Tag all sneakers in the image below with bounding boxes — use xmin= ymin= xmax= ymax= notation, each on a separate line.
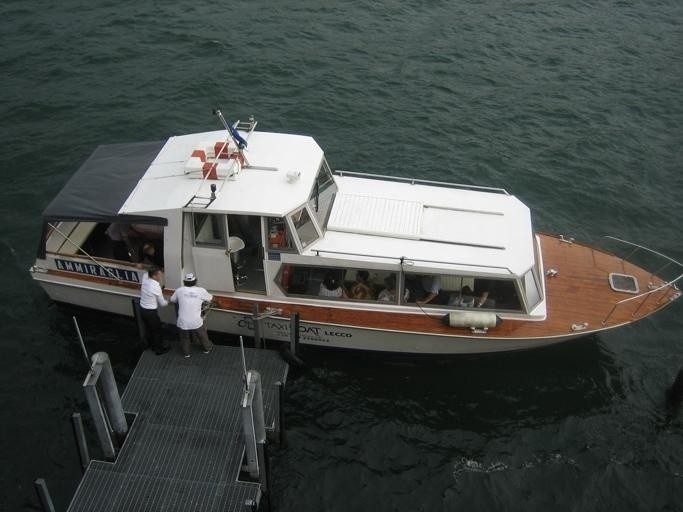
xmin=203 ymin=343 xmax=213 ymax=353
xmin=184 ymin=354 xmax=192 ymax=358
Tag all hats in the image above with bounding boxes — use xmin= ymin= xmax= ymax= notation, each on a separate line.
xmin=183 ymin=273 xmax=196 ymax=282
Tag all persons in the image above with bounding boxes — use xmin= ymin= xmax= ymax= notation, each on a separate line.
xmin=318 ymin=268 xmax=515 ymax=309
xmin=170 ymin=273 xmax=213 ymax=357
xmin=139 ymin=266 xmax=176 ymax=355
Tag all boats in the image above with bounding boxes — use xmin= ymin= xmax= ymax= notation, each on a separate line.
xmin=23 ymin=107 xmax=682 ymax=359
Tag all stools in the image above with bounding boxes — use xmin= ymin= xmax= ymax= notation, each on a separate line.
xmin=228 ymin=236 xmax=247 ymax=287
